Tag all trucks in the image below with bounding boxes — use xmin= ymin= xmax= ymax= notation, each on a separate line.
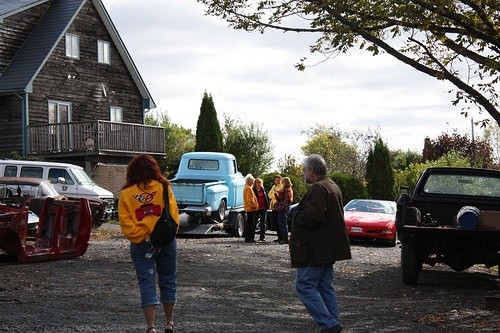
xmin=168 ymin=151 xmax=246 ymax=237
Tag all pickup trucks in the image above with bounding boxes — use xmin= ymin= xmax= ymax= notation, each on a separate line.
xmin=395 ymin=167 xmax=500 ymax=285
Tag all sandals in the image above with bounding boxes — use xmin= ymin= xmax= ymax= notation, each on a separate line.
xmin=163 ymin=323 xmax=173 ymax=333
xmin=145 ymin=327 xmax=157 ymax=333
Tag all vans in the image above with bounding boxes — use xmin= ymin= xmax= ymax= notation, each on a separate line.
xmin=0 ymin=159 xmax=116 ymax=266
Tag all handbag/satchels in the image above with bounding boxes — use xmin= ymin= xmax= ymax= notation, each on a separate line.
xmin=274 ymin=202 xmax=287 ymax=212
xmin=150 ymin=212 xmax=178 ymax=247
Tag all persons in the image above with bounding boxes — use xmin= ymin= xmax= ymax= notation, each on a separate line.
xmin=242 ymin=174 xmax=259 ymax=245
xmin=268 ymin=175 xmax=284 ymax=241
xmin=118 ymin=154 xmax=179 ymax=333
xmin=252 ymin=178 xmax=270 ymax=241
xmin=289 ymin=154 xmax=352 ymax=333
xmin=273 ymin=177 xmax=293 ymax=243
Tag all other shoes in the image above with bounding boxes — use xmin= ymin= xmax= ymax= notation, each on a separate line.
xmin=274 ymin=239 xmax=281 ymax=242
xmin=320 ymin=324 xmax=342 ymax=333
xmin=279 ymin=240 xmax=289 ymax=244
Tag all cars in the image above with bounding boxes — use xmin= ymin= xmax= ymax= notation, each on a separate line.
xmin=343 ymin=198 xmax=401 ymax=247
xmin=255 ymin=202 xmax=299 ymax=234
